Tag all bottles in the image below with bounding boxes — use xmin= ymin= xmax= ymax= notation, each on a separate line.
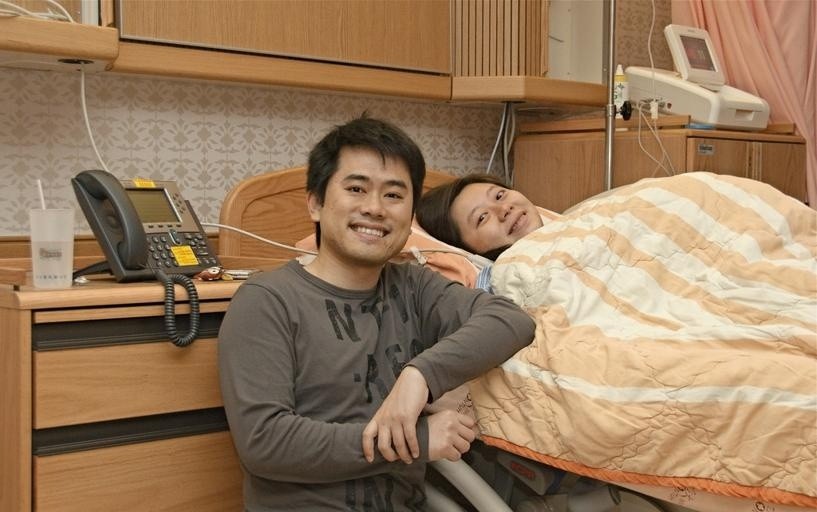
xmin=612 ymin=63 xmax=628 ymax=134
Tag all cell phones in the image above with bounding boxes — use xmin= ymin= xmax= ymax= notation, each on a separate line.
xmin=223 ymin=268 xmax=260 ymax=280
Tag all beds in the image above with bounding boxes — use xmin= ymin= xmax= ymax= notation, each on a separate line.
xmin=210 ymin=162 xmax=817 ymax=512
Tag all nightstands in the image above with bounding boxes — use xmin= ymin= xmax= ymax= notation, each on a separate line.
xmin=0 ymin=254 xmax=252 ymax=511
xmin=514 ymin=118 xmax=807 ymax=213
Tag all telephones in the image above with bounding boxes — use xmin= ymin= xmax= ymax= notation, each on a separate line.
xmin=71 ymin=169 xmax=224 ymax=283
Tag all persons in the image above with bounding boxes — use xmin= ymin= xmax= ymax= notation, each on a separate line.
xmin=216 ymin=113 xmax=537 ymax=512
xmin=414 ymin=174 xmax=544 ymax=258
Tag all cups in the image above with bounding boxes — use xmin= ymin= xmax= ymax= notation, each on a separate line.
xmin=26 ymin=206 xmax=78 ymax=291
